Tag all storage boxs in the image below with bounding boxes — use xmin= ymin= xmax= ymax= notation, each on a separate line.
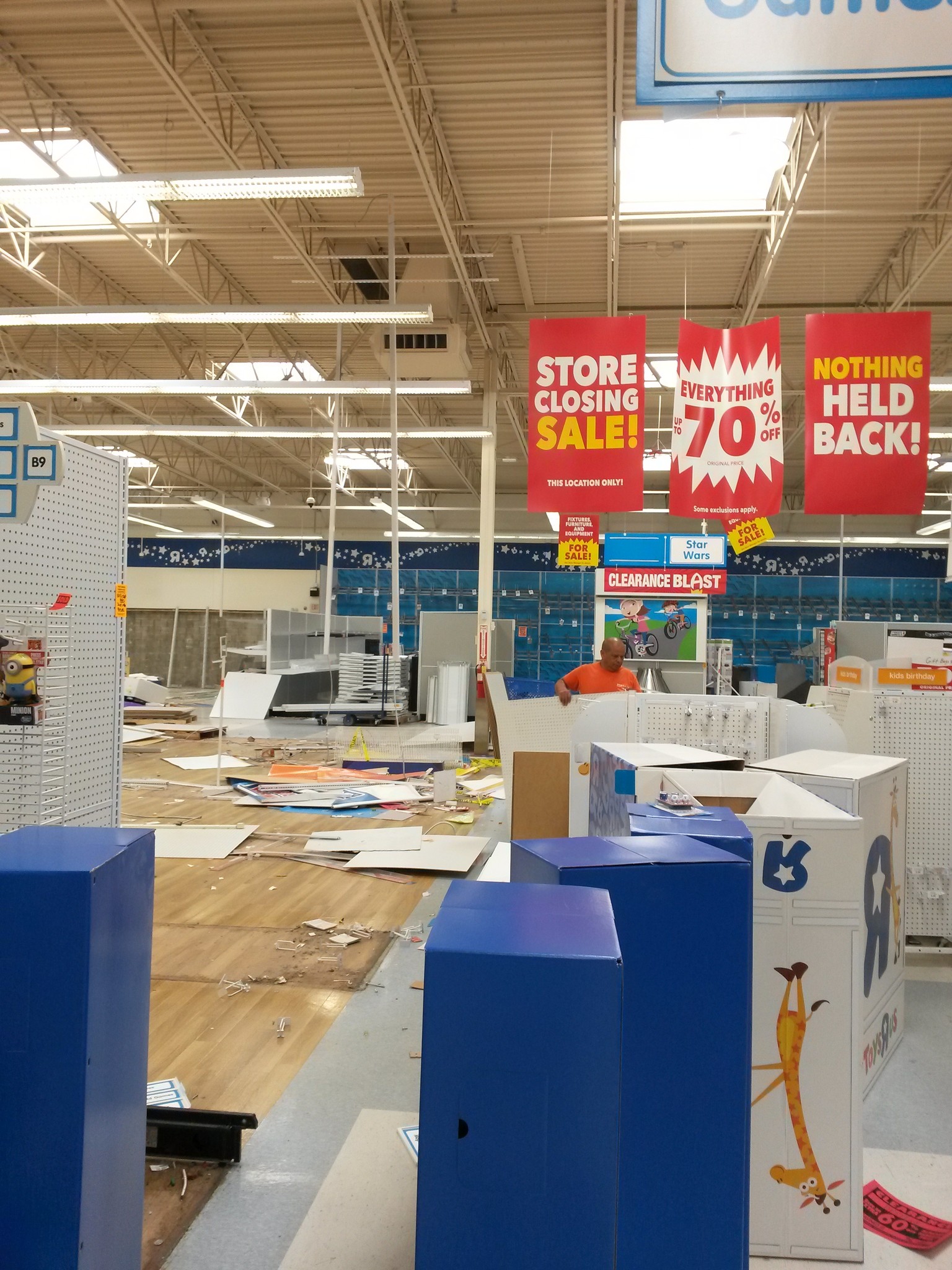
xmin=415 ymin=742 xmax=909 ymax=1270
xmin=0 ymin=823 xmax=156 ymax=1269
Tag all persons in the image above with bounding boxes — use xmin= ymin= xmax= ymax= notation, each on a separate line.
xmin=554 ymin=638 xmax=642 ymax=706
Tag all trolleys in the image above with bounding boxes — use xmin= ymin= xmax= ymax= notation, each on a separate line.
xmin=316 ymin=641 xmax=389 ymax=726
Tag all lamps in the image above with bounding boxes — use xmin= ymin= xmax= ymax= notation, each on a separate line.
xmin=0 ymin=169 xmax=367 ymax=206
xmin=0 ymin=304 xmax=493 ymax=440
xmin=368 ymin=496 xmax=426 ymax=532
xmin=188 ymin=494 xmax=275 ymax=529
xmin=916 ymin=518 xmax=952 ymax=537
xmin=127 ymin=512 xmax=183 ymax=533
xmin=928 ymin=376 xmax=952 ymax=392
xmin=928 ymin=426 xmax=952 ymax=438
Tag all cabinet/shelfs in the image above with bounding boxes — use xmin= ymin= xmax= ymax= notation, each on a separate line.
xmin=333 ymin=586 xmax=952 ymax=664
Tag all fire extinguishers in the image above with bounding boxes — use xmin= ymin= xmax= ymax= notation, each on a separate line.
xmin=475 ymin=662 xmax=486 ymax=699
